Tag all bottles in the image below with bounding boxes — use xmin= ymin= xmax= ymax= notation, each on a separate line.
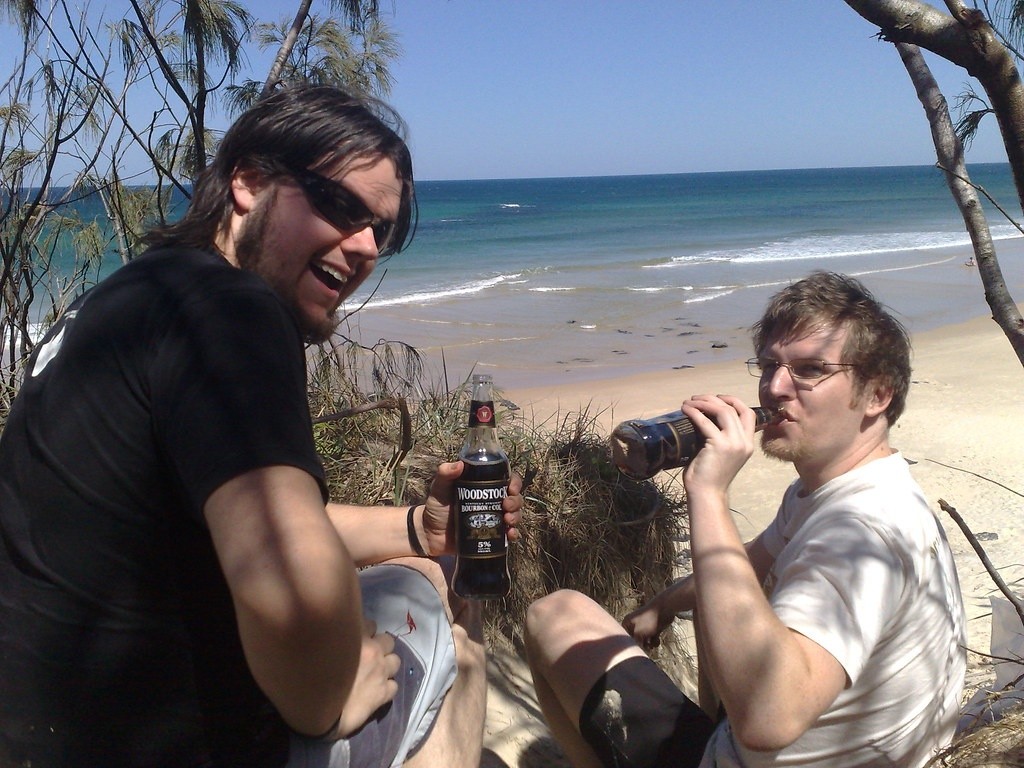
xmin=609 ymin=404 xmax=778 ymax=484
xmin=450 ymin=373 xmax=514 ymax=603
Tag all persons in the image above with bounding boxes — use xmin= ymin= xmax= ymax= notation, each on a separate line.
xmin=522 ymin=270 xmax=967 ymax=768
xmin=0 ymin=85 xmax=522 ymax=768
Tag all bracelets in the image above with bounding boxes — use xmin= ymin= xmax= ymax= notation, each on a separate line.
xmin=290 ymin=713 xmax=342 ymax=739
xmin=407 ymin=504 xmax=428 ymax=558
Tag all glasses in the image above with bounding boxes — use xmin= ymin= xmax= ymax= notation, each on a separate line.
xmin=277 ymin=158 xmax=399 ymax=251
xmin=745 ymin=356 xmax=853 ymax=379
